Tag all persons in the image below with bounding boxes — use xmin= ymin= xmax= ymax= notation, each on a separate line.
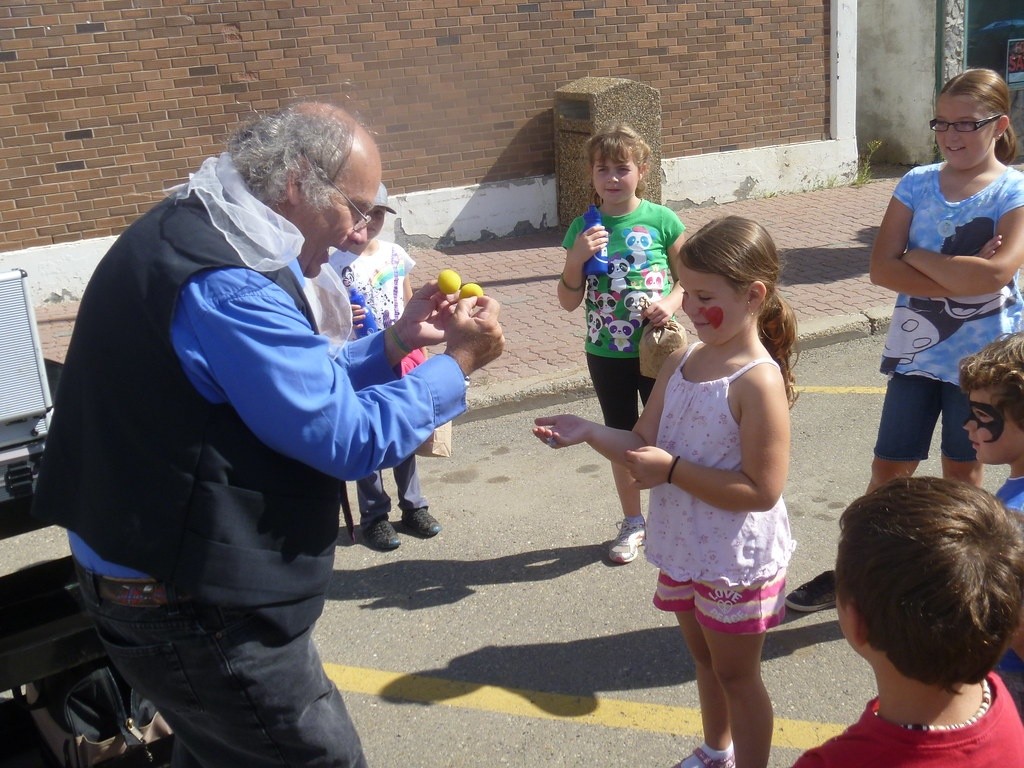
xmin=32 ymin=100 xmax=506 ymax=768
xmin=327 ymin=182 xmax=443 ymax=553
xmin=783 ymin=69 xmax=1024 ymax=613
xmin=557 ymin=124 xmax=687 ymax=564
xmin=531 ymin=213 xmax=799 ymax=768
xmin=789 ymin=333 xmax=1024 ymax=768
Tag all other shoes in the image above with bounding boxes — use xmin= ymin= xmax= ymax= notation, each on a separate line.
xmin=672 ymin=747 xmax=735 ymax=768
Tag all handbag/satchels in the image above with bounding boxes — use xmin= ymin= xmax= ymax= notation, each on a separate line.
xmin=26 ymin=662 xmax=174 ymax=768
xmin=415 ymin=419 xmax=452 ymax=457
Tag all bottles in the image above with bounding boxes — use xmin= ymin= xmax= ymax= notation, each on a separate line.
xmin=582 ymin=205 xmax=608 ymax=274
xmin=349 ymin=287 xmax=376 ymax=341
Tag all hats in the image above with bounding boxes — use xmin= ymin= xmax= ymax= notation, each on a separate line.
xmin=373 ymin=182 xmax=396 ymax=216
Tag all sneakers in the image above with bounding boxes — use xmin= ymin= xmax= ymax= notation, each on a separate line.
xmin=785 ymin=571 xmax=837 ymax=610
xmin=362 ymin=519 xmax=401 ymax=549
xmin=608 ymin=519 xmax=647 ymax=562
xmin=402 ymin=508 xmax=442 ymax=536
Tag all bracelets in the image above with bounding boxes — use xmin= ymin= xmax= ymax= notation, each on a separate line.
xmin=447 ymin=354 xmax=471 ymax=391
xmin=560 ymin=273 xmax=583 ymax=292
xmin=667 ymin=455 xmax=681 ymax=485
xmin=387 ymin=327 xmax=413 ymax=356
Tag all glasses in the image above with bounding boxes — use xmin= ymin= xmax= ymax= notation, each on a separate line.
xmin=300 ymin=148 xmax=372 ymax=232
xmin=930 ymin=113 xmax=1004 ymax=132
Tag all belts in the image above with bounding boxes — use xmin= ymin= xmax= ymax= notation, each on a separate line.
xmin=73 ymin=563 xmax=167 ymax=610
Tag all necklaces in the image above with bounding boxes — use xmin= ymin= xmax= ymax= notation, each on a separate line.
xmin=900 ymin=676 xmax=993 ymax=732
